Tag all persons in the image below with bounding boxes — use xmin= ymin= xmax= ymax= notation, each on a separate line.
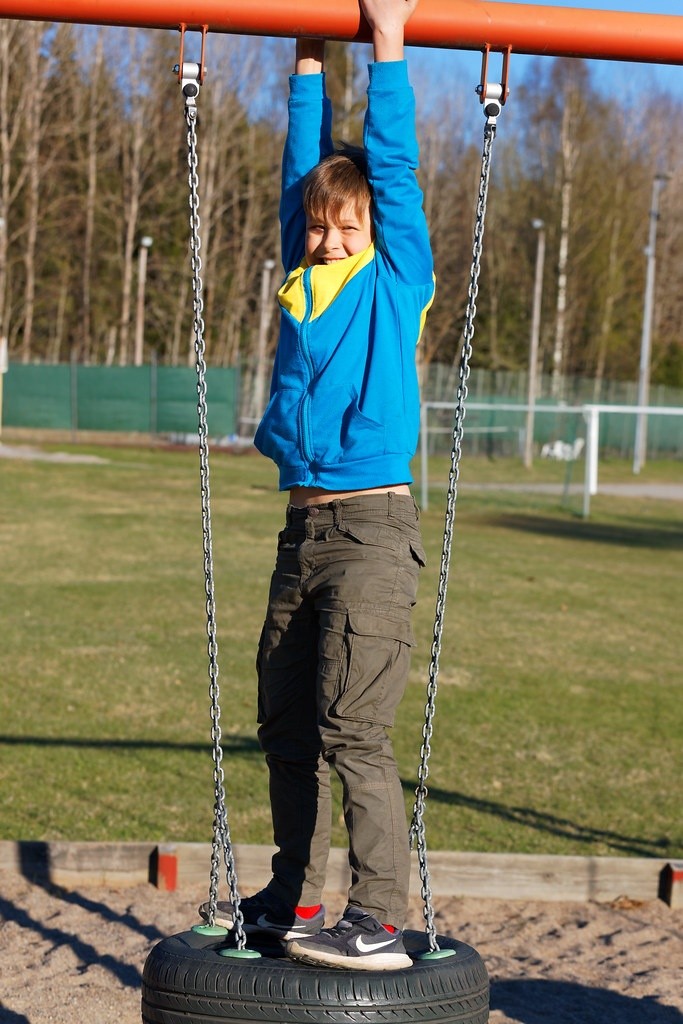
xmin=199 ymin=0 xmax=441 ymax=971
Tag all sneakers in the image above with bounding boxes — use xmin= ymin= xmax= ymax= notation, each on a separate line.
xmin=286 ymin=906 xmax=412 ymax=972
xmin=198 ymin=883 xmax=325 ymax=941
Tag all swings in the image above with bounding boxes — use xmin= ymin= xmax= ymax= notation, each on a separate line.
xmin=142 ymin=23 xmax=514 ymax=1024
